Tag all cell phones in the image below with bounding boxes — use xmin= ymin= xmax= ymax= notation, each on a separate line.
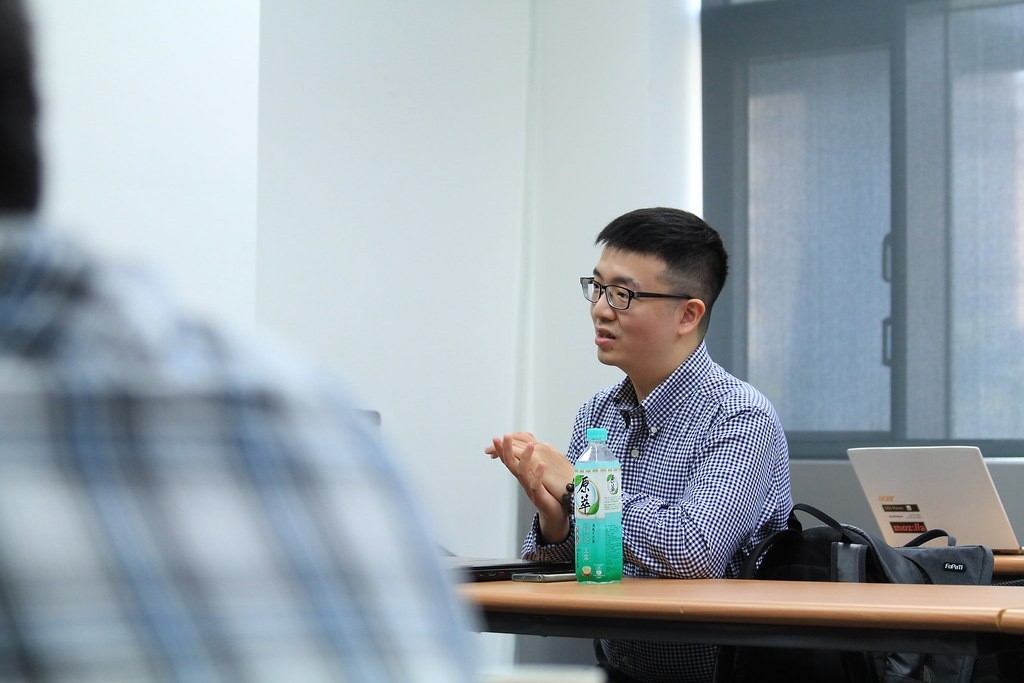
xmin=512 ymin=573 xmax=576 ymax=582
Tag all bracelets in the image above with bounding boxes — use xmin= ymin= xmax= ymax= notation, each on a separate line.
xmin=559 ymin=475 xmax=578 ymax=512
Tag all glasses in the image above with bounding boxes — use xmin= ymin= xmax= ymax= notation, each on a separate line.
xmin=580 ymin=276 xmax=707 ymax=312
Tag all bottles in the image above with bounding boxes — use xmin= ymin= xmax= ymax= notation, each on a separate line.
xmin=574 ymin=429 xmax=623 ymax=583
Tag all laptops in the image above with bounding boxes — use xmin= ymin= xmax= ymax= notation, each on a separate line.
xmin=442 ymin=555 xmax=575 ymax=584
xmin=846 ymin=446 xmax=1024 ymax=555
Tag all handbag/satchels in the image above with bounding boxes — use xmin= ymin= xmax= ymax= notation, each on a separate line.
xmin=713 ymin=503 xmax=994 ymax=683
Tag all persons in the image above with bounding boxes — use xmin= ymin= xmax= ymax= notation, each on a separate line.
xmin=0 ymin=0 xmax=483 ymax=682
xmin=484 ymin=206 xmax=795 ymax=683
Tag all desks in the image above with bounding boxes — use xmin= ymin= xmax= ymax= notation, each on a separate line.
xmin=454 ymin=578 xmax=1023 ymax=682
